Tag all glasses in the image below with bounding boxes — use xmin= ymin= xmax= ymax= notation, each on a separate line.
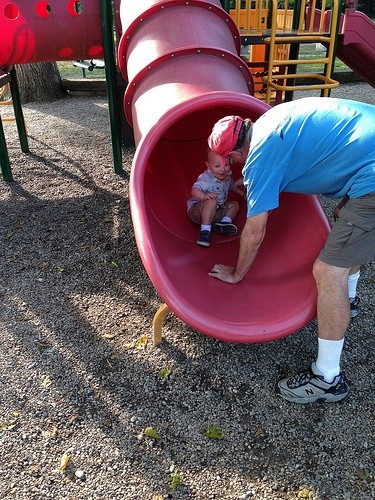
xmin=227 ymin=156 xmax=235 ymax=166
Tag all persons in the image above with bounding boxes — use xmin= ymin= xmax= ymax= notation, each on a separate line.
xmin=186 ymin=149 xmax=248 ymax=247
xmin=209 ymin=97 xmax=375 ymax=405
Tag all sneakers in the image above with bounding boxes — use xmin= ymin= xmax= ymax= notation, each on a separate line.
xmin=275 ymin=362 xmax=348 ymax=402
xmin=197 ymin=230 xmax=211 ymax=247
xmin=348 ymin=297 xmax=361 ymax=318
xmin=213 ymin=221 xmax=238 ymax=236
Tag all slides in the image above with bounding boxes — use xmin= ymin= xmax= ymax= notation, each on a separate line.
xmin=112 ymin=0 xmax=335 ymax=344
xmin=304 ymin=6 xmax=375 ymax=90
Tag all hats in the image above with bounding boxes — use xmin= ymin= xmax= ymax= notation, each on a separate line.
xmin=207 ymin=115 xmax=243 ymax=167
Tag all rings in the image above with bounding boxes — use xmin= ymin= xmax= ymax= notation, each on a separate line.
xmin=217 ymin=269 xmax=218 ymax=272
